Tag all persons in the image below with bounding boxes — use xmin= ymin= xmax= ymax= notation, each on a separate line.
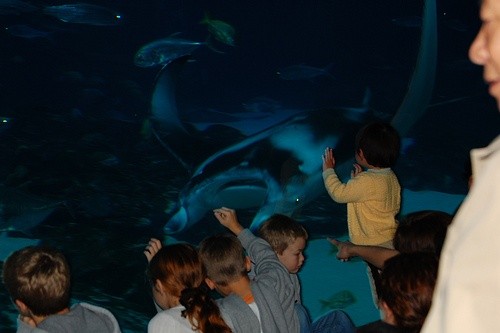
xmin=326 ymin=208 xmax=452 ymax=284
xmin=417 ymin=1 xmax=499 ymax=332
xmin=254 ymin=212 xmax=359 ymax=332
xmin=352 ymin=255 xmax=436 ymax=332
xmin=320 ymin=122 xmax=403 ymax=320
xmin=0 ymin=243 xmax=121 ymax=332
xmin=146 ymin=242 xmax=232 ymax=332
xmin=142 ymin=205 xmax=300 ymax=332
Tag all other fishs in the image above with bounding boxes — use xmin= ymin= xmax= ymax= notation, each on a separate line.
xmin=1 ymin=0 xmax=468 ymax=262
